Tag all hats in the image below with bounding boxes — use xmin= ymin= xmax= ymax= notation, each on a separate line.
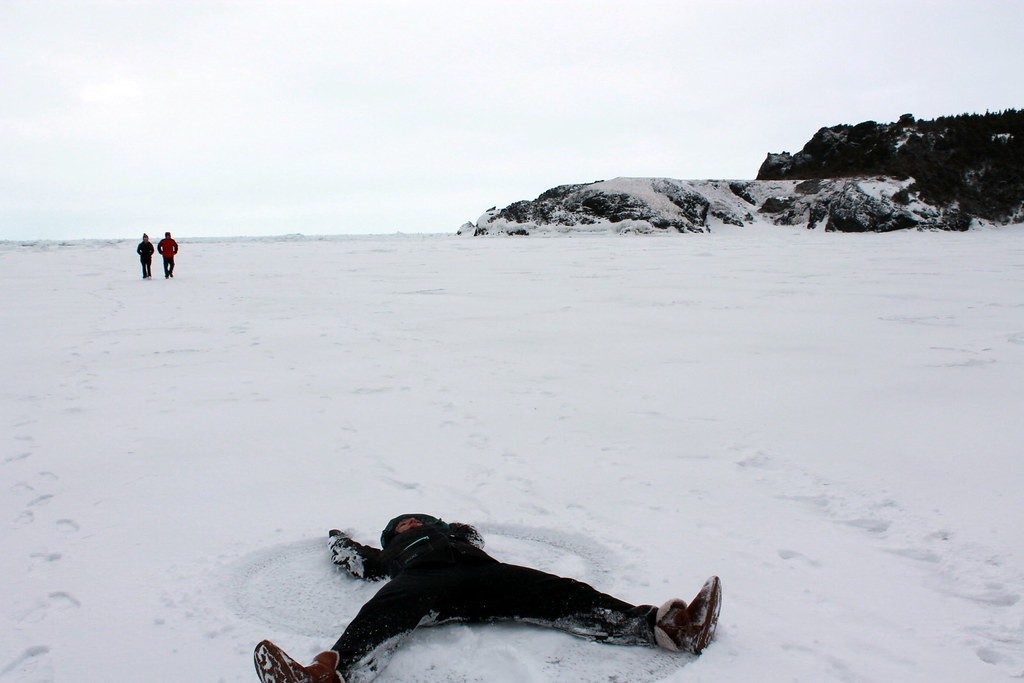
xmin=142 ymin=233 xmax=147 ymax=237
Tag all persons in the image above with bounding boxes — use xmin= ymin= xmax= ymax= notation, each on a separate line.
xmin=255 ymin=514 xmax=722 ymax=683
xmin=158 ymin=232 xmax=178 ymax=279
xmin=137 ymin=234 xmax=155 ymax=279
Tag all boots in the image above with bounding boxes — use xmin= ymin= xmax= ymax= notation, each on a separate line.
xmin=254 ymin=640 xmax=348 ymax=683
xmin=656 ymin=576 xmax=722 ymax=653
